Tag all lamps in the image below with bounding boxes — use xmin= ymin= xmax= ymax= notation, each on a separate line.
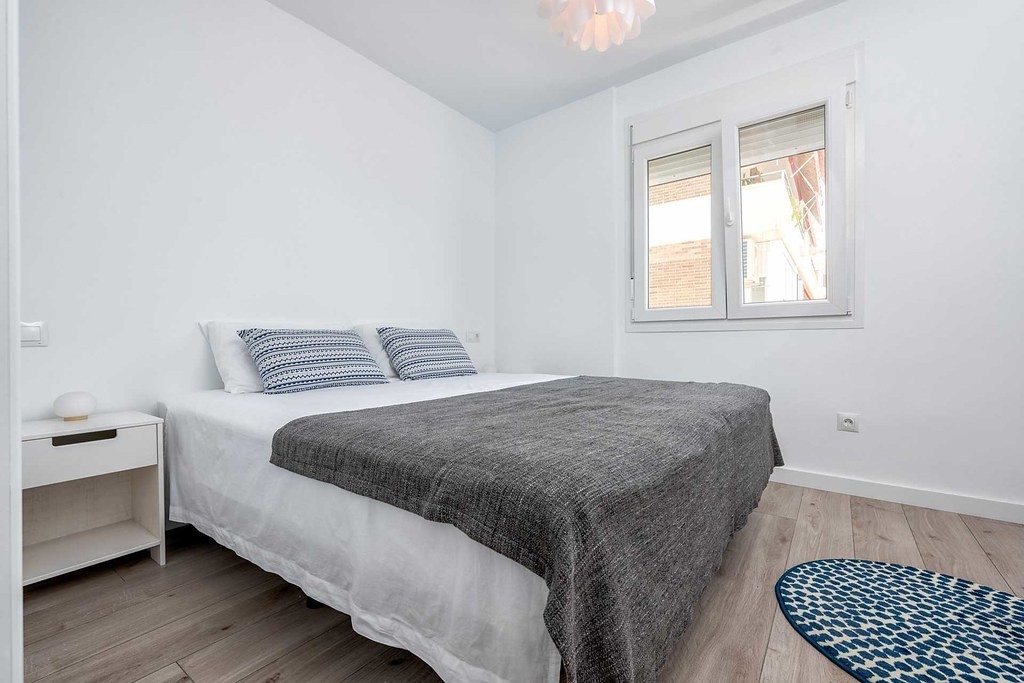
xmin=55 ymin=390 xmax=99 ymax=422
xmin=536 ymin=0 xmax=657 ymax=52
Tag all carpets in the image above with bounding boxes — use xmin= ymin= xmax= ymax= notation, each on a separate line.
xmin=774 ymin=558 xmax=1024 ymax=683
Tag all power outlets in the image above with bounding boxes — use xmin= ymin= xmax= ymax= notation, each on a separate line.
xmin=836 ymin=412 xmax=860 ymax=433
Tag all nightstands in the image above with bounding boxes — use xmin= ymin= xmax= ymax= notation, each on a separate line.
xmin=21 ymin=411 xmax=166 ymax=592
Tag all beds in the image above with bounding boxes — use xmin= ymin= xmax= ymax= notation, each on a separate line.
xmin=153 ymin=313 xmax=786 ymax=683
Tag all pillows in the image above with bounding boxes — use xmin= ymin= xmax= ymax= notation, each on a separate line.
xmin=236 ymin=329 xmax=389 ymax=393
xmin=356 ymin=322 xmax=465 ymax=378
xmin=376 ymin=327 xmax=478 ymax=382
xmin=198 ymin=320 xmax=357 ymax=394
